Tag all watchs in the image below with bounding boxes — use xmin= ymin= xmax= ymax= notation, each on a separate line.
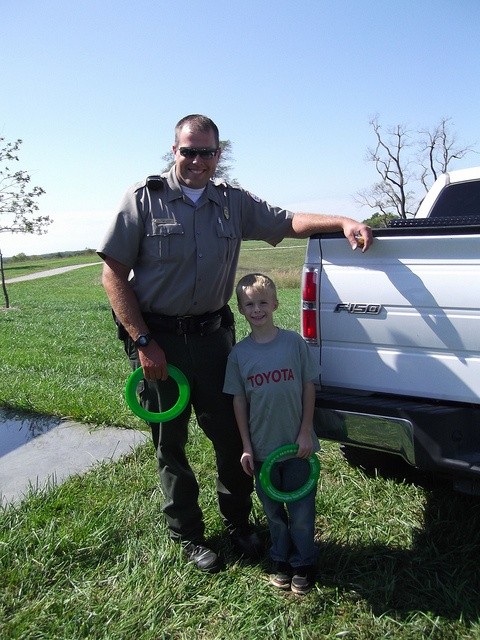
xmin=134 ymin=334 xmax=154 ymax=351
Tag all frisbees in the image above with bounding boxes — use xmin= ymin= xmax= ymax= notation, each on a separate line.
xmin=259 ymin=443 xmax=320 ymax=502
xmin=124 ymin=361 xmax=190 ymax=423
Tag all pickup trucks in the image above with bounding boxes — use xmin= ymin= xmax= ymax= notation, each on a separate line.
xmin=302 ymin=167 xmax=480 ymax=494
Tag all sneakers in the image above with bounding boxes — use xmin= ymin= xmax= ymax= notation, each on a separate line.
xmin=291 ymin=566 xmax=313 ymax=594
xmin=270 ymin=561 xmax=292 ymax=588
xmin=190 ymin=546 xmax=221 ymax=572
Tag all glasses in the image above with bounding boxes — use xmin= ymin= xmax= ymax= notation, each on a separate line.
xmin=178 ymin=147 xmax=221 ymax=159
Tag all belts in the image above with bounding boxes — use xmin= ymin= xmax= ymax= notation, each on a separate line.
xmin=143 ymin=314 xmax=222 ymax=337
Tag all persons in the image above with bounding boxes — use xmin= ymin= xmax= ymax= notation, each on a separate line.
xmin=222 ymin=273 xmax=323 ymax=597
xmin=95 ymin=114 xmax=373 ymax=573
